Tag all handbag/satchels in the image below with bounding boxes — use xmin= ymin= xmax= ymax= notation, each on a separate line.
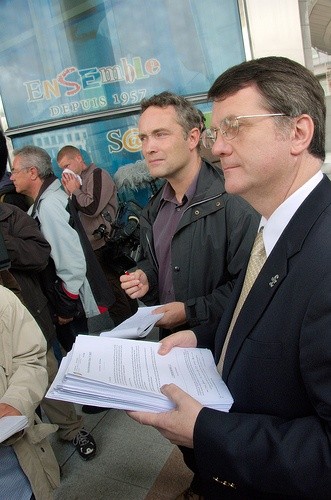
xmin=92 ymin=198 xmax=150 ymax=265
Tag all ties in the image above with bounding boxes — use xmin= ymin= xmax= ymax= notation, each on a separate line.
xmin=216 ymin=229 xmax=267 ymax=378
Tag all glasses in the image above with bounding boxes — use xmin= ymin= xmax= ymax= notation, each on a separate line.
xmin=198 ymin=113 xmax=288 ymax=150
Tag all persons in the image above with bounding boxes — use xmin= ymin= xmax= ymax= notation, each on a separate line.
xmin=0 ymin=203 xmax=97 ymax=458
xmin=9 ymin=145 xmax=119 ymax=414
xmin=0 ymin=284 xmax=63 ymax=500
xmin=0 ymin=91 xmax=259 ymax=340
xmin=124 ymin=55 xmax=331 ymax=500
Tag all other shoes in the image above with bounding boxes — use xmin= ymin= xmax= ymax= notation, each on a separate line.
xmin=81 ymin=404 xmax=109 ymax=414
xmin=70 ymin=427 xmax=96 ymax=461
